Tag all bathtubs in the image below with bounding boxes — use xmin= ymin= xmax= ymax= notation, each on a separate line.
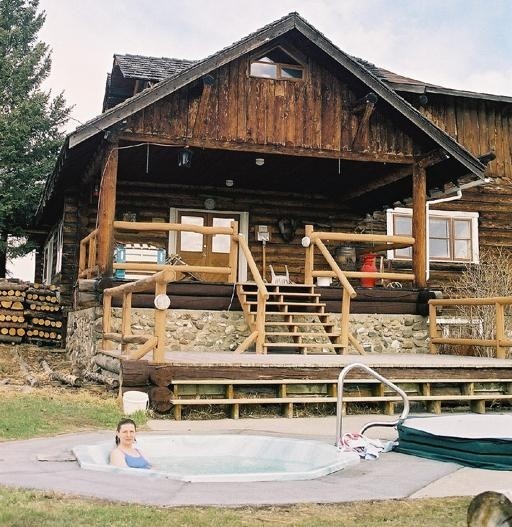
xmin=71 ymin=434 xmax=363 ymax=482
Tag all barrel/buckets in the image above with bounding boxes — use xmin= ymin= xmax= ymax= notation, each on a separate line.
xmin=122 ymin=390 xmax=149 ymax=417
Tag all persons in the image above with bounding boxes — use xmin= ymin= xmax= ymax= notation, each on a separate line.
xmin=109 ymin=417 xmax=159 ymax=471
